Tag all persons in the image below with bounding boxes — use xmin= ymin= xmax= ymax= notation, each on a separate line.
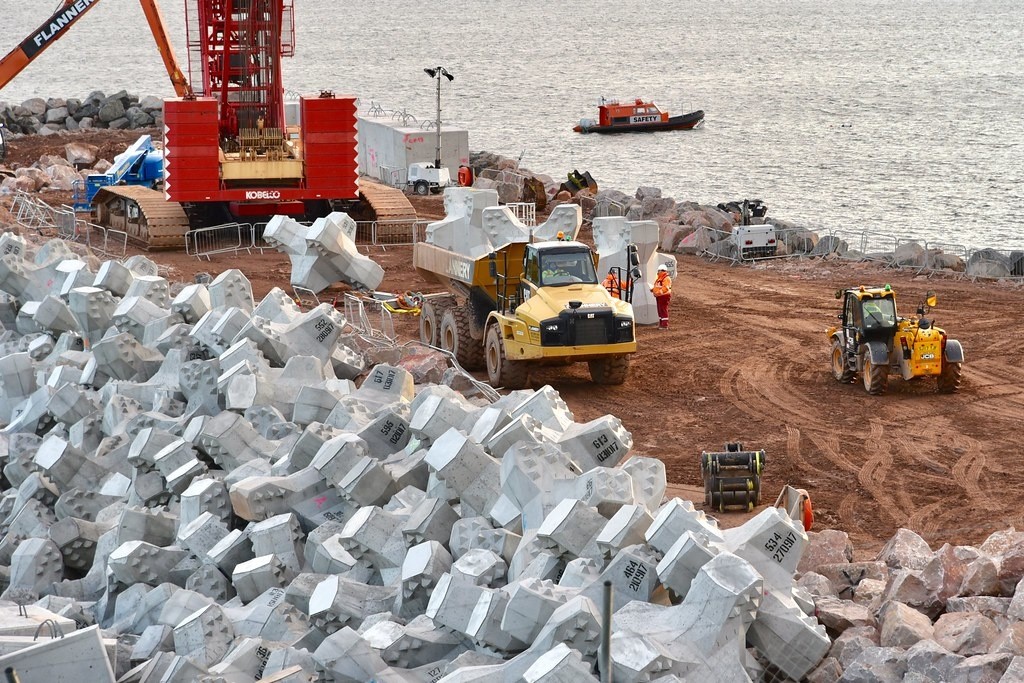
xmin=542 ymin=261 xmax=570 ymax=282
xmin=650 ymin=264 xmax=672 ymax=331
xmin=600 ymin=269 xmax=632 ymax=299
xmin=863 ymin=300 xmax=881 ymax=318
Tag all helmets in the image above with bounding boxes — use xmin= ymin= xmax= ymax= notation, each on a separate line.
xmin=608 ymin=269 xmax=616 ymax=274
xmin=658 ymin=264 xmax=668 ymax=271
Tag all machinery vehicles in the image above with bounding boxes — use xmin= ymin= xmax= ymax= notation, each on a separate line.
xmin=71 ymin=135 xmax=164 ymax=213
xmin=412 ymin=231 xmax=641 ymax=389
xmin=717 ymin=198 xmax=777 ymax=265
xmin=0 ymin=0 xmax=418 ymax=252
xmin=407 ymin=65 xmax=484 ymax=197
xmin=824 ymin=284 xmax=964 ymax=396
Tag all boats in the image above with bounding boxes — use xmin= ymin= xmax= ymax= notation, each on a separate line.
xmin=572 ymin=95 xmax=705 ymax=135
